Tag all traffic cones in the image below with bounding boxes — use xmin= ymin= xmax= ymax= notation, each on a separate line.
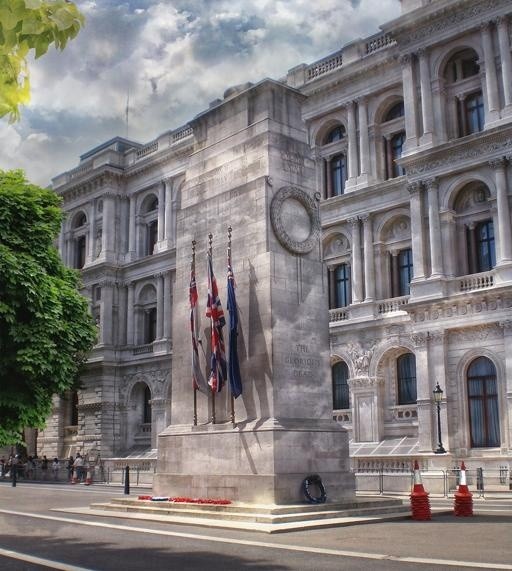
xmin=85 ymin=468 xmax=93 ymax=486
xmin=71 ymin=468 xmax=79 ymax=484
xmin=410 ymin=461 xmax=432 ymax=521
xmin=454 ymin=461 xmax=473 ymax=517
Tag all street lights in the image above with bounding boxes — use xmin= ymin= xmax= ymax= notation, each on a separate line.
xmin=432 ymin=382 xmax=448 ymax=453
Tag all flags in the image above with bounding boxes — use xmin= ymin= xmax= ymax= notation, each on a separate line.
xmin=226 ymin=265 xmax=243 ymax=399
xmin=189 ymin=261 xmax=206 ymax=394
xmin=206 ymin=256 xmax=226 ymax=392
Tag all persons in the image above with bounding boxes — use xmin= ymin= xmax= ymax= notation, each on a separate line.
xmin=0 ymin=453 xmax=85 ymax=484
